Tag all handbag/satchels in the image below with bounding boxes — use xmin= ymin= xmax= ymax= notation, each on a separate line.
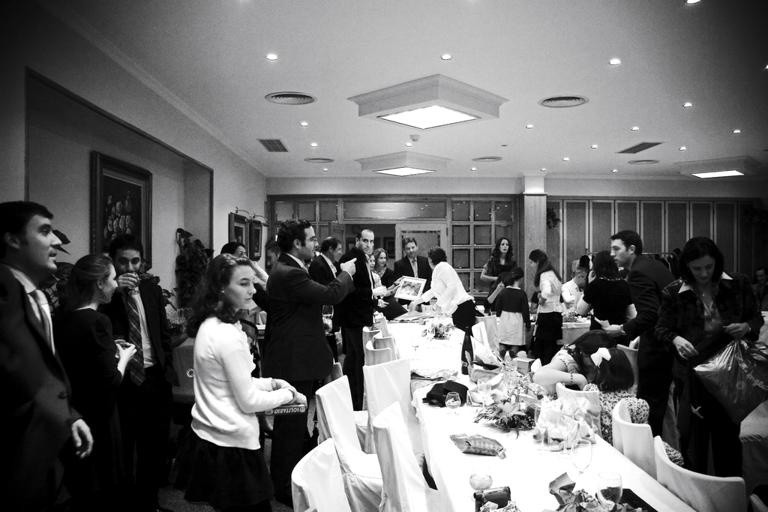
xmin=422 ymin=378 xmax=469 ymax=406
xmin=694 ymin=334 xmax=768 ymax=425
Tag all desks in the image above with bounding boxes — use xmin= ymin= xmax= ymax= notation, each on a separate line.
xmin=529 ymin=313 xmax=591 ymax=346
xmin=382 ymin=310 xmax=694 ymax=511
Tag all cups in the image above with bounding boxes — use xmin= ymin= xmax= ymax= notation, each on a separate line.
xmin=596 ymin=473 xmax=622 ymax=512
xmin=446 ymin=392 xmax=460 ymax=415
xmin=321 ymin=303 xmax=335 ymax=336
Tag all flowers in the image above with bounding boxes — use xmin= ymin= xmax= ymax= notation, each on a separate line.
xmin=463 ymin=351 xmax=557 ymax=430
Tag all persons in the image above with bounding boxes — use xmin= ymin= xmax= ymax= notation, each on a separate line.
xmin=529 ymin=249 xmax=565 ymax=365
xmin=577 ymin=250 xmax=637 ymax=331
xmin=338 ymin=229 xmax=390 ymax=411
xmin=367 ymin=254 xmax=405 ymax=297
xmin=373 ymin=247 xmax=395 ymax=288
xmin=220 ymin=241 xmax=269 ymax=311
xmin=184 ymin=252 xmax=309 ymax=512
xmin=393 ymin=237 xmax=432 ymax=306
xmin=307 ymin=237 xmax=342 ymax=362
xmin=102 ymin=235 xmax=181 ymax=511
xmin=265 ymin=239 xmax=281 ymax=271
xmin=0 ymin=199 xmax=96 ymax=512
xmin=575 ymin=347 xmax=683 ymax=462
xmin=533 ymin=332 xmax=617 ymax=393
xmin=496 ymin=267 xmax=532 ymax=357
xmin=752 ymin=265 xmax=768 ymax=310
xmin=63 ymin=252 xmax=137 ymax=512
xmin=262 ymin=219 xmax=357 ymax=512
xmin=480 ymin=237 xmax=517 ymax=315
xmin=655 ymin=235 xmax=765 ymax=479
xmin=595 ymin=230 xmax=678 ymax=440
xmin=561 ymin=254 xmax=591 ymax=314
xmin=408 ymin=246 xmax=484 ymax=376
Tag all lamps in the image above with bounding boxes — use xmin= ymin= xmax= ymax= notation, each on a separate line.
xmin=671 ymin=154 xmax=768 ymax=186
xmin=347 ymin=73 xmax=508 ymax=131
xmin=355 ymin=151 xmax=455 ymax=180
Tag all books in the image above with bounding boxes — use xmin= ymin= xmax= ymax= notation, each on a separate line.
xmin=264 ymin=405 xmax=303 ymax=412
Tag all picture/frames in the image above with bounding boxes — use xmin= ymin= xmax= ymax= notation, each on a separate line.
xmin=86 ymin=149 xmax=154 ymax=275
xmin=391 ymin=275 xmax=428 ymax=302
xmin=226 ymin=212 xmax=252 ymax=260
xmin=249 ymin=218 xmax=265 ymax=261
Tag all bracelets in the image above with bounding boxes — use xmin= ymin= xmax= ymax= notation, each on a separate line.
xmin=570 ymin=372 xmax=575 ymax=387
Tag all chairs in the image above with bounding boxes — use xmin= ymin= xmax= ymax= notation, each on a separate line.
xmin=610 ymin=400 xmax=658 ymax=479
xmin=291 ymin=437 xmax=353 ymax=512
xmin=362 ymin=357 xmax=424 ymax=439
xmin=361 ymin=322 xmax=387 ymax=341
xmin=365 ymin=336 xmax=399 ymax=367
xmin=315 ymin=375 xmax=384 ymax=512
xmin=554 ymin=380 xmax=602 ymax=439
xmin=652 ymin=433 xmax=750 ymax=511
xmin=372 ymin=400 xmax=454 ymax=511
